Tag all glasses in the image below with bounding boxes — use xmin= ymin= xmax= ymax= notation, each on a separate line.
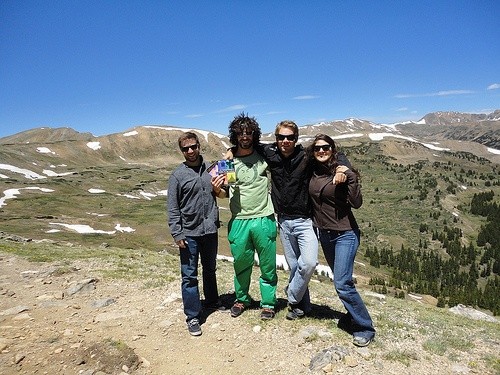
xmin=235 ymin=131 xmax=254 ymax=136
xmin=312 ymin=145 xmax=332 ymax=151
xmin=276 ymin=134 xmax=295 ymax=141
xmin=181 ymin=141 xmax=198 ymax=152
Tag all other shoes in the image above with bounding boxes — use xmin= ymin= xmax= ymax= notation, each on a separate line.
xmin=287 ymin=303 xmax=305 ymax=319
xmin=353 ymin=335 xmax=373 ymax=347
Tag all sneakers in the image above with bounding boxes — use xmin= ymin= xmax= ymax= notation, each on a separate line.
xmin=205 ymin=300 xmax=228 ymax=310
xmin=231 ymin=301 xmax=245 ymax=317
xmin=187 ymin=317 xmax=201 ymax=335
xmin=260 ymin=308 xmax=275 ymax=319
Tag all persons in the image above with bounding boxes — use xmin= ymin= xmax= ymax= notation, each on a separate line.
xmin=223 ymin=120 xmax=352 ymax=320
xmin=167 ymin=131 xmax=229 ymax=336
xmin=308 ymin=133 xmax=376 ymax=347
xmin=210 ymin=112 xmax=278 ymax=320
